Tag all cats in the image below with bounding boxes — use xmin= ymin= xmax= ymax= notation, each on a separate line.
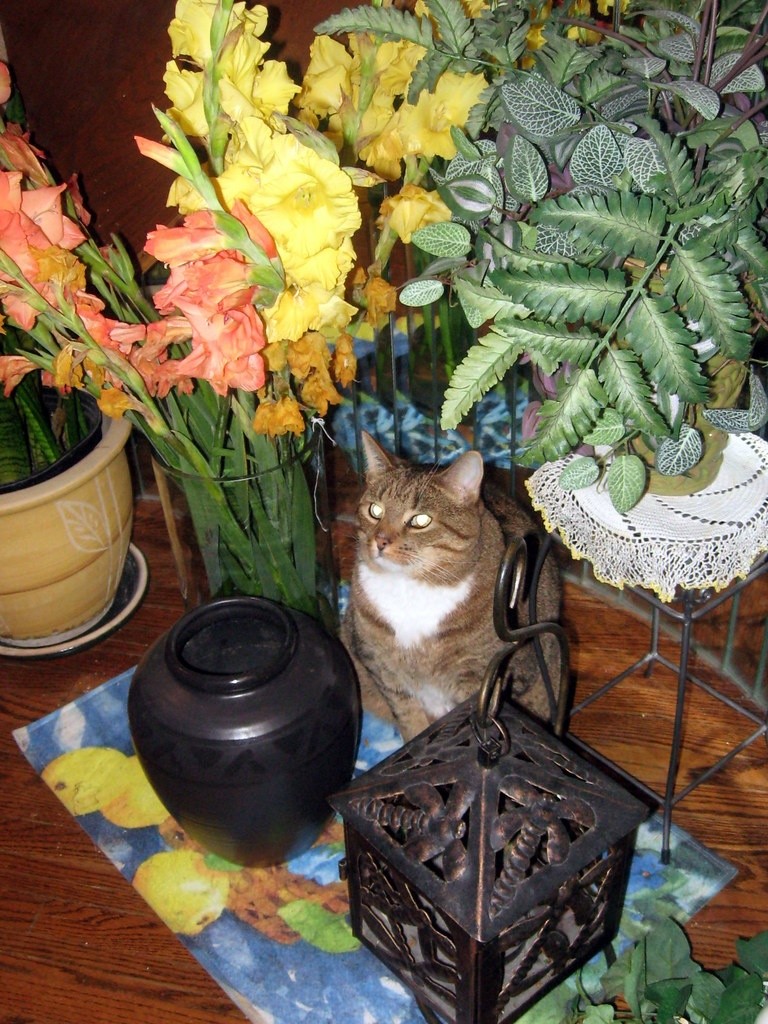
xmin=338 ymin=428 xmax=561 ymax=746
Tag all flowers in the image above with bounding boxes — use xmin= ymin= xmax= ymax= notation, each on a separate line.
xmin=0 ymin=0 xmax=647 ymax=607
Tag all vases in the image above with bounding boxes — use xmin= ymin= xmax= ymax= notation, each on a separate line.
xmin=0 ymin=394 xmax=147 ymax=662
xmin=149 ymin=408 xmax=342 ymax=634
xmin=336 ymin=181 xmax=529 ymax=473
